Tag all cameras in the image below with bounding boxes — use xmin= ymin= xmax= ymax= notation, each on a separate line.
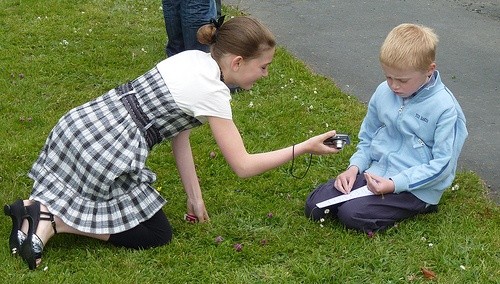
xmin=324 ymin=134 xmax=350 ymax=149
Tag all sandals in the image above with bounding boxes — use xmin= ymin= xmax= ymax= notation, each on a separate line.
xmin=20 ymin=201 xmax=58 ymax=270
xmin=4 ymin=198 xmax=28 ymax=257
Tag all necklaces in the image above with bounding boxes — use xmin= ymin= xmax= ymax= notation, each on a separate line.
xmin=211 ymin=55 xmax=226 ymax=83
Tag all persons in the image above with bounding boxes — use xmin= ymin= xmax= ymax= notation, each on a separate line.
xmin=304 ymin=23 xmax=469 ymax=231
xmin=161 ymin=0 xmax=218 ymax=58
xmin=3 ymin=16 xmax=339 ymax=270
